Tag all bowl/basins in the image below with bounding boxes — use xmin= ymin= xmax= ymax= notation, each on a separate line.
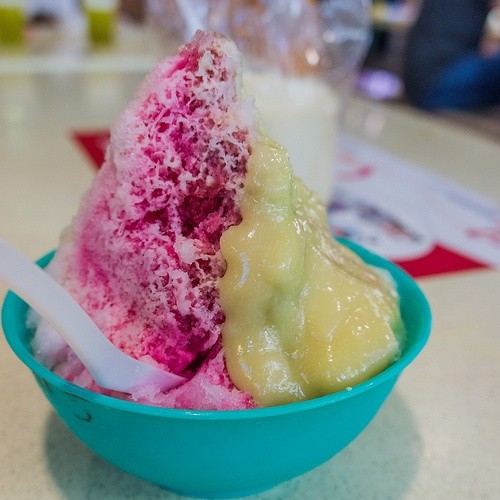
xmin=0 ymin=235 xmax=432 ymax=500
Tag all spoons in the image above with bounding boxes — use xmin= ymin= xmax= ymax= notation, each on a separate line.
xmin=0 ymin=239 xmax=186 ymax=395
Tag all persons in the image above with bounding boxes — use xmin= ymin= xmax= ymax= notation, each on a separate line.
xmin=402 ymin=0 xmax=500 ymax=110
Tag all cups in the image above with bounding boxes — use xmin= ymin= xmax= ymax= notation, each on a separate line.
xmin=0 ymin=0 xmax=25 ymax=54
xmin=85 ymin=0 xmax=121 ymax=50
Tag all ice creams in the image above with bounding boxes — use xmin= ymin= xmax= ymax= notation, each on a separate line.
xmin=24 ymin=28 xmax=405 ymax=412
